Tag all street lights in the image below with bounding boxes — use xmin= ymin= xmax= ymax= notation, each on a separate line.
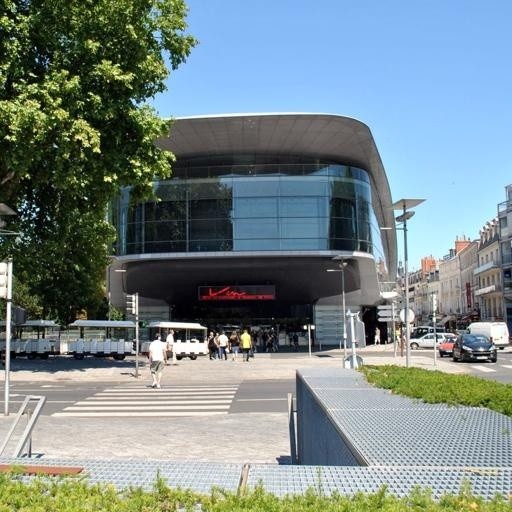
xmin=303 ymin=323 xmax=316 ymax=356
xmin=327 ymin=252 xmax=354 ymax=370
xmin=379 ymin=195 xmax=425 ymax=371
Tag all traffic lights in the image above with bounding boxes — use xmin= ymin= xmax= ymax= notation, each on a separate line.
xmin=0 ymin=256 xmax=11 ymax=301
xmin=126 ymin=292 xmax=137 ymax=316
xmin=139 ymin=319 xmax=147 ymax=328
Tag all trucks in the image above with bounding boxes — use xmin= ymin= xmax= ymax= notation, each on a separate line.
xmin=466 ymin=322 xmax=510 ymax=350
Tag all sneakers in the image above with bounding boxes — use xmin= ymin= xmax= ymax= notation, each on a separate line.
xmin=209 ymin=356 xmax=249 ymax=362
xmin=152 ymin=380 xmax=161 ymax=389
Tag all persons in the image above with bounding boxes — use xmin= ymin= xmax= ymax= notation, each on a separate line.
xmin=166 ymin=329 xmax=178 ymax=365
xmin=208 ymin=327 xmax=300 ymax=362
xmin=366 ymin=326 xmax=406 ymax=349
xmin=148 ymin=333 xmax=167 ymax=389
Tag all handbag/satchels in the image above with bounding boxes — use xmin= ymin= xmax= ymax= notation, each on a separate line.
xmin=248 ymin=349 xmax=254 ymax=358
xmin=166 ymin=351 xmax=173 ymax=359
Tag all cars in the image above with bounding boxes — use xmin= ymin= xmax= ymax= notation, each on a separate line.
xmin=399 ymin=325 xmax=467 ymax=360
xmin=451 ymin=332 xmax=498 ymax=363
